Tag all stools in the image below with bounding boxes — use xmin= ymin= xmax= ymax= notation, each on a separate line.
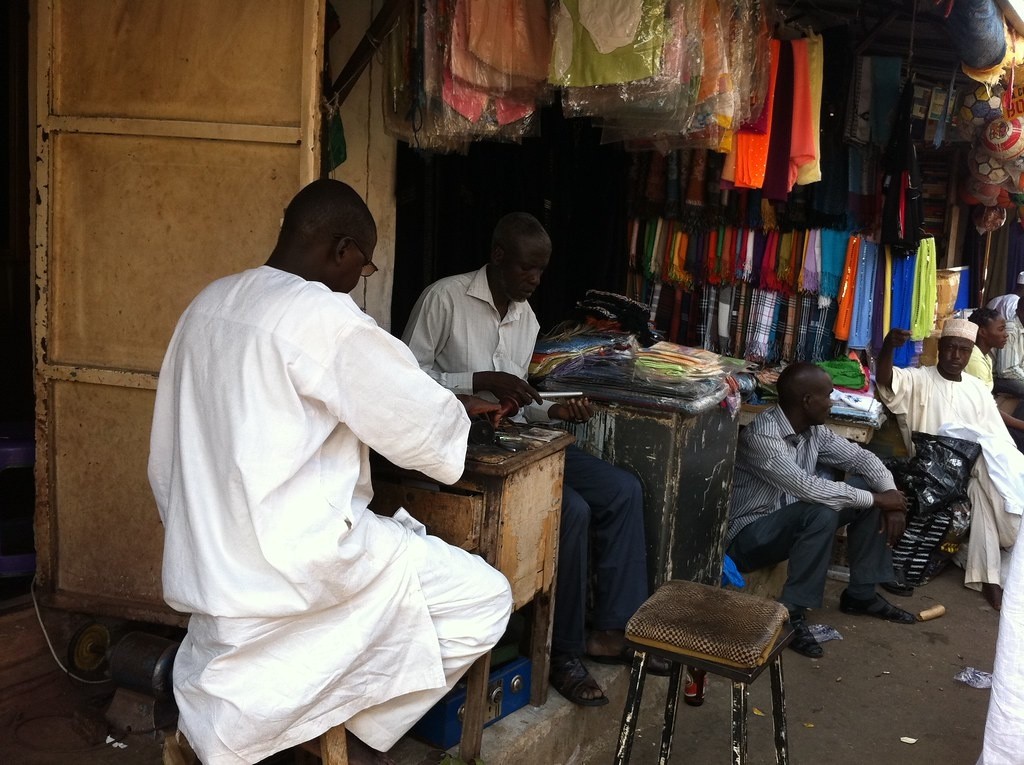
xmin=613 ymin=579 xmax=795 ymax=765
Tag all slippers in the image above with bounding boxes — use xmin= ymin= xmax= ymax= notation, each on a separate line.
xmin=588 ymin=644 xmax=672 ymax=676
xmin=549 ymin=658 xmax=610 ymax=706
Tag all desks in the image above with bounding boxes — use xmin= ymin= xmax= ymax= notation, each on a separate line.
xmin=367 ymin=411 xmax=579 ymax=765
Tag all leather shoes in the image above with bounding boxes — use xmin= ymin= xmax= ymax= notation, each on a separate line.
xmin=787 ymin=613 xmax=824 ymax=657
xmin=839 ymin=589 xmax=916 ymax=624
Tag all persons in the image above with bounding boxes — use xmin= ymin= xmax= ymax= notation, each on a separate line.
xmin=145 ymin=179 xmax=515 ymax=764
xmin=723 ymin=365 xmax=916 ymax=660
xmin=961 ymin=306 xmax=1024 ymax=453
xmin=987 ymin=270 xmax=1024 ymax=321
xmin=875 ymin=318 xmax=1024 ymax=615
xmin=987 ymin=297 xmax=1024 ymax=404
xmin=398 ymin=212 xmax=676 ymax=710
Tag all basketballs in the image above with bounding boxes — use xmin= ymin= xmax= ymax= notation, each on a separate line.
xmin=997 ymin=186 xmax=1018 ymax=209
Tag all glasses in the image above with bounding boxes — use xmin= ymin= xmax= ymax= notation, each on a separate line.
xmin=335 ymin=234 xmax=378 ymax=277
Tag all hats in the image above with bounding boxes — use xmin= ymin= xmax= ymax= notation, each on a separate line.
xmin=1017 ymin=271 xmax=1024 ymax=284
xmin=941 ymin=319 xmax=979 ymax=343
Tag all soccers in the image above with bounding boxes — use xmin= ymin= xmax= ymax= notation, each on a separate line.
xmin=957 ymin=81 xmax=1024 ymax=232
